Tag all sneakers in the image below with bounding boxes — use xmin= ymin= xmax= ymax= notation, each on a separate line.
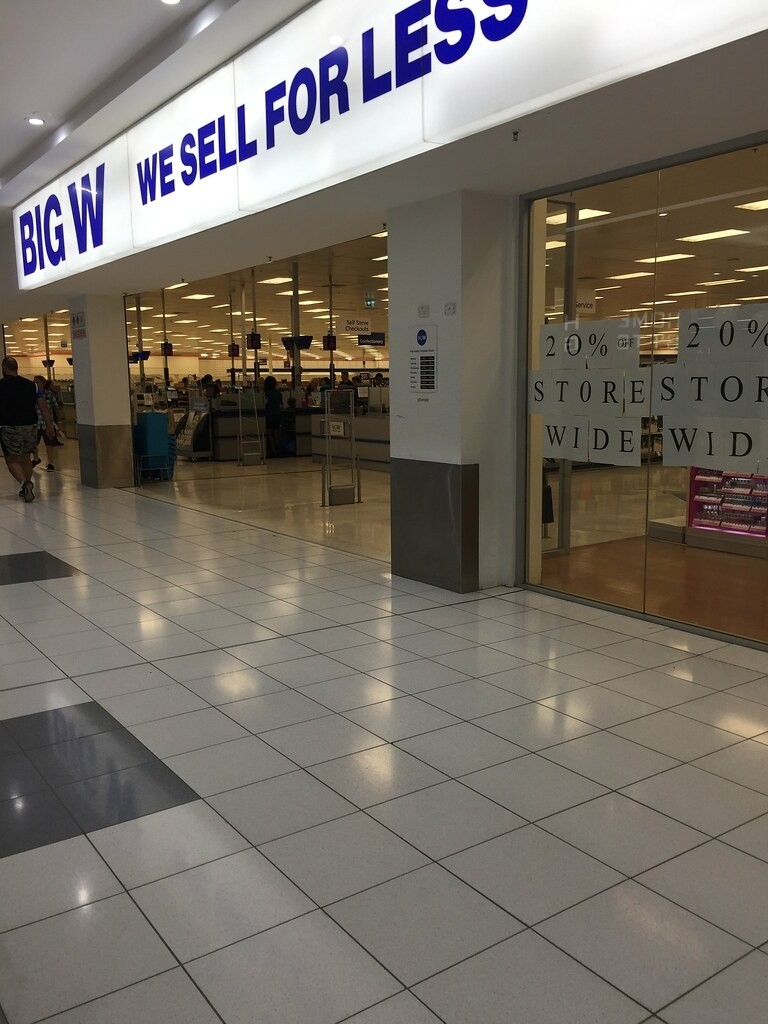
xmin=31 ymin=458 xmax=41 ymax=470
xmin=46 ymin=463 xmax=55 ymax=472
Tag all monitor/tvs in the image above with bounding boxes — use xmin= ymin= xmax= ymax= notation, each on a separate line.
xmin=355 ymin=385 xmax=371 ymax=398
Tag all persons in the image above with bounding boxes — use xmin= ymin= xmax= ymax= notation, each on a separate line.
xmin=321 ymin=377 xmax=330 ymax=385
xmin=375 ymin=373 xmax=383 ymax=385
xmin=31 ymin=376 xmax=61 ymax=472
xmin=338 ymin=370 xmax=358 ymax=417
xmin=46 ymin=380 xmax=64 ymax=446
xmin=0 ymin=357 xmax=55 ymax=503
xmin=202 ymin=374 xmax=213 ymax=385
xmin=265 ymin=376 xmax=281 ymax=458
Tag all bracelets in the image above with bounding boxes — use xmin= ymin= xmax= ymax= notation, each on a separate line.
xmin=57 ymin=421 xmax=62 ymax=424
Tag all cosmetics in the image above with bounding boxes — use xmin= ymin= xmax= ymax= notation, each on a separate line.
xmin=695 ymin=468 xmax=768 ymax=527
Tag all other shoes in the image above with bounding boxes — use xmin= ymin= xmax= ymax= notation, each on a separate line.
xmin=52 ymin=438 xmax=63 ymax=447
xmin=18 ymin=479 xmax=35 ymax=503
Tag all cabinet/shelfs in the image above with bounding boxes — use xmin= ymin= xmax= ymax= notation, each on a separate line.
xmin=685 ymin=466 xmax=768 ymax=560
